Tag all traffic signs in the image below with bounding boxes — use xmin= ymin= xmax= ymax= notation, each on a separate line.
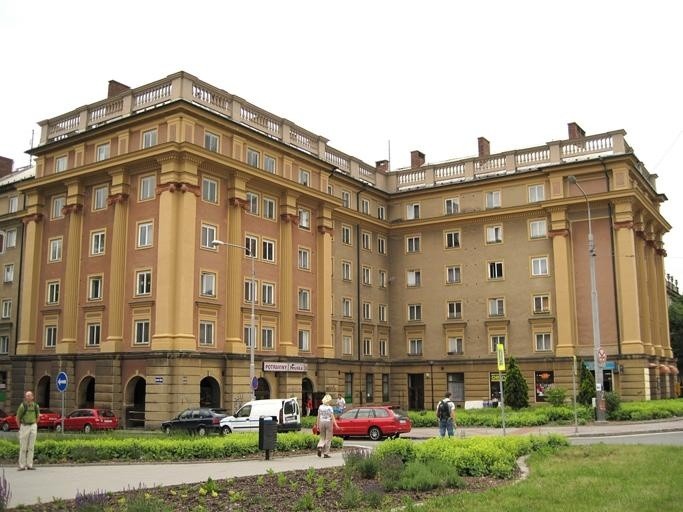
xmin=597 ymin=349 xmax=606 ymax=364
xmin=55 ymin=373 xmax=68 ymax=392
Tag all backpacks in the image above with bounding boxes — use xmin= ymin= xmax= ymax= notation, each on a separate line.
xmin=437 ymin=400 xmax=452 ymax=422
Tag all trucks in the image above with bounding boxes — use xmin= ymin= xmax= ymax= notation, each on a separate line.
xmin=219 ymin=398 xmax=300 ymax=436
xmin=0 ymin=409 xmax=61 ymax=432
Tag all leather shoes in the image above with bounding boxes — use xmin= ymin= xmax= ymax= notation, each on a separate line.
xmin=18 ymin=467 xmax=24 ymax=470
xmin=28 ymin=466 xmax=34 ymax=469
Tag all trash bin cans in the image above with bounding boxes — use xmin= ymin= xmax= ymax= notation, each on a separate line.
xmin=259 ymin=415 xmax=277 ymax=450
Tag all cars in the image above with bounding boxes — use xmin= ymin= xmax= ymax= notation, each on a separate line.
xmin=159 ymin=408 xmax=228 ymax=436
xmin=312 ymin=406 xmax=411 ymax=441
xmin=53 ymin=409 xmax=117 ymax=433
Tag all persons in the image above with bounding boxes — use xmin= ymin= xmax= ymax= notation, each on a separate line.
xmin=337 ymin=394 xmax=346 ymax=414
xmin=16 ymin=390 xmax=40 ymax=472
xmin=306 ymin=396 xmax=313 ymax=416
xmin=317 ymin=395 xmax=339 ymax=458
xmin=435 ymin=391 xmax=457 ymax=438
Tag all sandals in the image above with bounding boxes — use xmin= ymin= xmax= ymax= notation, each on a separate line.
xmin=324 ymin=455 xmax=331 ymax=458
xmin=317 ymin=448 xmax=321 ymax=457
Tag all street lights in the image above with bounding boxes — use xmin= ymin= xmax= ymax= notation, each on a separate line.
xmin=426 ymin=362 xmax=435 ymax=412
xmin=209 ymin=240 xmax=257 ymax=401
xmin=567 ymin=177 xmax=605 ymax=422
